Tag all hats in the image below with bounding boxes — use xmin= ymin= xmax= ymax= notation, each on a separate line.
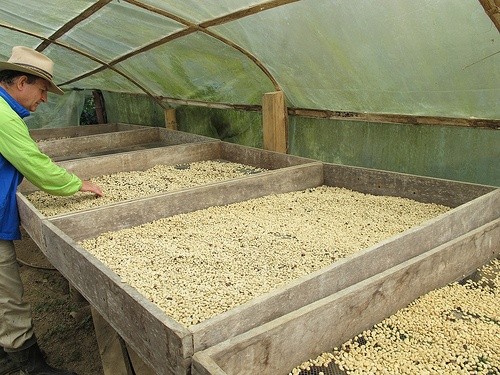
xmin=0 ymin=46 xmax=64 ymax=95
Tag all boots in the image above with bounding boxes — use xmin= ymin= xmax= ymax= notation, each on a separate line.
xmin=0 ymin=334 xmax=77 ymax=375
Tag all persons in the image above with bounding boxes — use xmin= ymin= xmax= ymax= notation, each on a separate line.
xmin=0 ymin=45 xmax=106 ymax=375
